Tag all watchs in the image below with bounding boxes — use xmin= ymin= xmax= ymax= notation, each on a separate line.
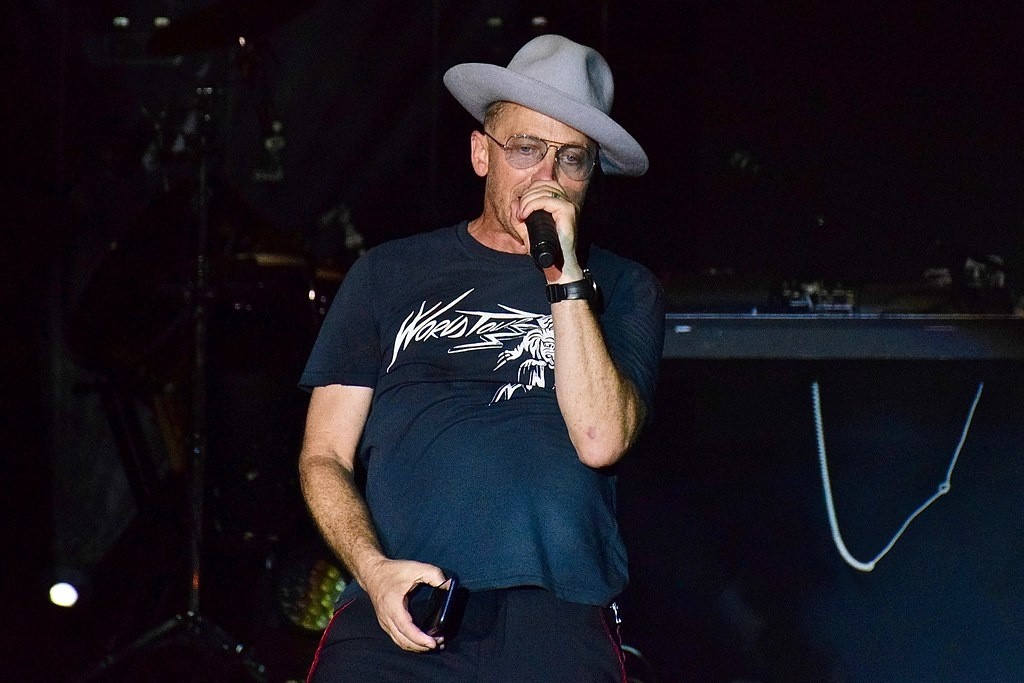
xmin=546 ymin=269 xmax=599 ymax=303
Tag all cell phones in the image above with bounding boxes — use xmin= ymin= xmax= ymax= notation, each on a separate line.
xmin=421 ymin=574 xmax=460 ymax=637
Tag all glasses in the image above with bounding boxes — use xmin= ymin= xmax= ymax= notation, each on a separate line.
xmin=476 ymin=128 xmax=600 ymax=181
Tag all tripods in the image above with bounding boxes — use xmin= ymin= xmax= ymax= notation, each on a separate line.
xmin=64 ymin=46 xmax=288 ymax=683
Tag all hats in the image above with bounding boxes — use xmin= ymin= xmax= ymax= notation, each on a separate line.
xmin=443 ymin=33 xmax=649 ymax=176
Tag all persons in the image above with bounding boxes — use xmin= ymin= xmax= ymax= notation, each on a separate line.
xmin=298 ymin=35 xmax=666 ymax=683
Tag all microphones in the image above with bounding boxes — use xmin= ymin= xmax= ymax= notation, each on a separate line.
xmin=524 ymin=209 xmax=560 ymax=268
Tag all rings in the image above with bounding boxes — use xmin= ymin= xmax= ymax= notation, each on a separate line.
xmin=552 ymin=192 xmax=560 ymax=198
